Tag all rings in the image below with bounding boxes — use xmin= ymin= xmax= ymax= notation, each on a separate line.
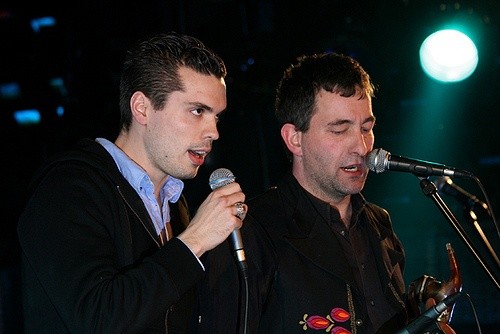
xmin=234 ymin=202 xmax=244 ymax=217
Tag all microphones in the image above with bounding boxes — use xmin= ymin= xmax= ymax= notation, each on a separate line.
xmin=399 ymin=291 xmax=462 ymax=334
xmin=366 ymin=148 xmax=472 ymax=178
xmin=434 ymin=175 xmax=489 ymax=213
xmin=208 ymin=168 xmax=248 ymax=280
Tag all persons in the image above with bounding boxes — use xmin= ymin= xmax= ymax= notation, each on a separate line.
xmin=16 ymin=31 xmax=429 ymax=334
